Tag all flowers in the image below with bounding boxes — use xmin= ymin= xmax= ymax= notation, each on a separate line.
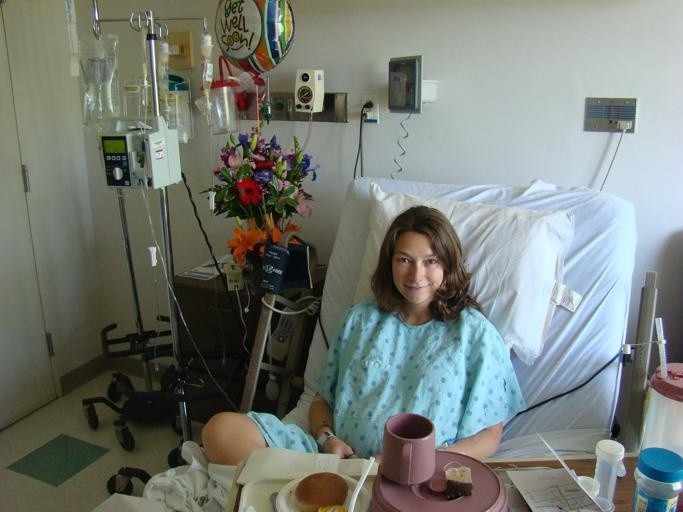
xmin=199 ymin=128 xmax=320 ymax=264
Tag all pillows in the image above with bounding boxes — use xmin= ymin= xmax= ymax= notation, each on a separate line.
xmin=356 ymin=182 xmax=574 ymax=361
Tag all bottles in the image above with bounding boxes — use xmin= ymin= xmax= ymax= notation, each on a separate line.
xmin=590 ymin=439 xmax=625 ymax=512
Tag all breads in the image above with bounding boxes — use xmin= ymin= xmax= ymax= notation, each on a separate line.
xmin=294 ymin=472 xmax=348 ymax=512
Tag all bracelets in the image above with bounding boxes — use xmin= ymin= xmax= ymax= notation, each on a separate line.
xmin=316 ymin=431 xmax=336 ymax=445
xmin=314 ymin=423 xmax=334 ymax=434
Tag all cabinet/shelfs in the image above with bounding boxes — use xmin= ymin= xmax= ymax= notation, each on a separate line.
xmin=170 ymin=264 xmax=328 ymax=424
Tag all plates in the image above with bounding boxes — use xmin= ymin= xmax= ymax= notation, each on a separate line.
xmin=275 ymin=471 xmax=371 ymax=512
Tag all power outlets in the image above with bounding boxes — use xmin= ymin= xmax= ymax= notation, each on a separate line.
xmin=585 ymin=97 xmax=635 ymax=135
xmin=360 ymin=95 xmax=379 ymax=123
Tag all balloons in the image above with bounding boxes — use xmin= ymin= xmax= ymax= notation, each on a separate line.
xmin=213 ymin=0 xmax=296 ymax=76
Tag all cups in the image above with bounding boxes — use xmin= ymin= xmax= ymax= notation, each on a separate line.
xmin=379 ymin=412 xmax=437 ymax=487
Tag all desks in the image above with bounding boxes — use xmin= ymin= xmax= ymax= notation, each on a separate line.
xmin=226 ymin=453 xmax=683 ymax=512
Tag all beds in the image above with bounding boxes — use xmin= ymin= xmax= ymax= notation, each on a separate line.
xmin=144 ymin=177 xmax=657 ymax=512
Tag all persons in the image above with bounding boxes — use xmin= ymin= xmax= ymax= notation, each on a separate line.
xmin=201 ymin=205 xmax=528 ymax=466
